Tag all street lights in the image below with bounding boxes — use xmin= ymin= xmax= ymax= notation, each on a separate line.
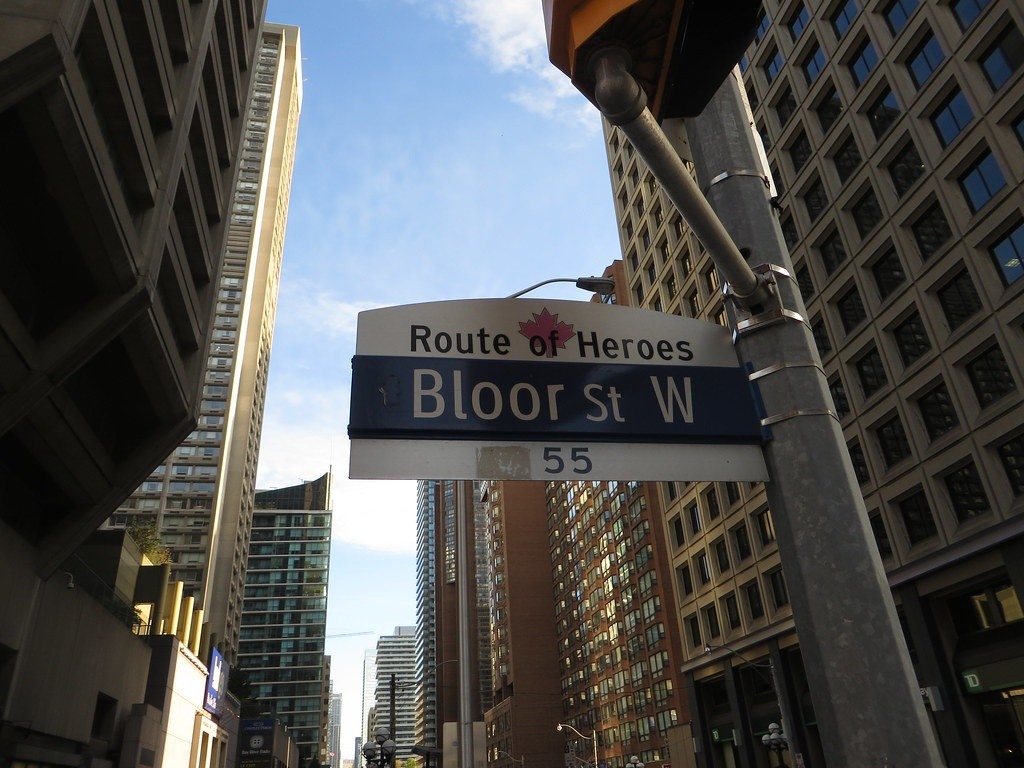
xmin=557 ymin=723 xmax=597 ymax=768
xmin=455 ymin=274 xmax=617 ymax=768
xmin=389 ymin=659 xmax=460 ymax=768
